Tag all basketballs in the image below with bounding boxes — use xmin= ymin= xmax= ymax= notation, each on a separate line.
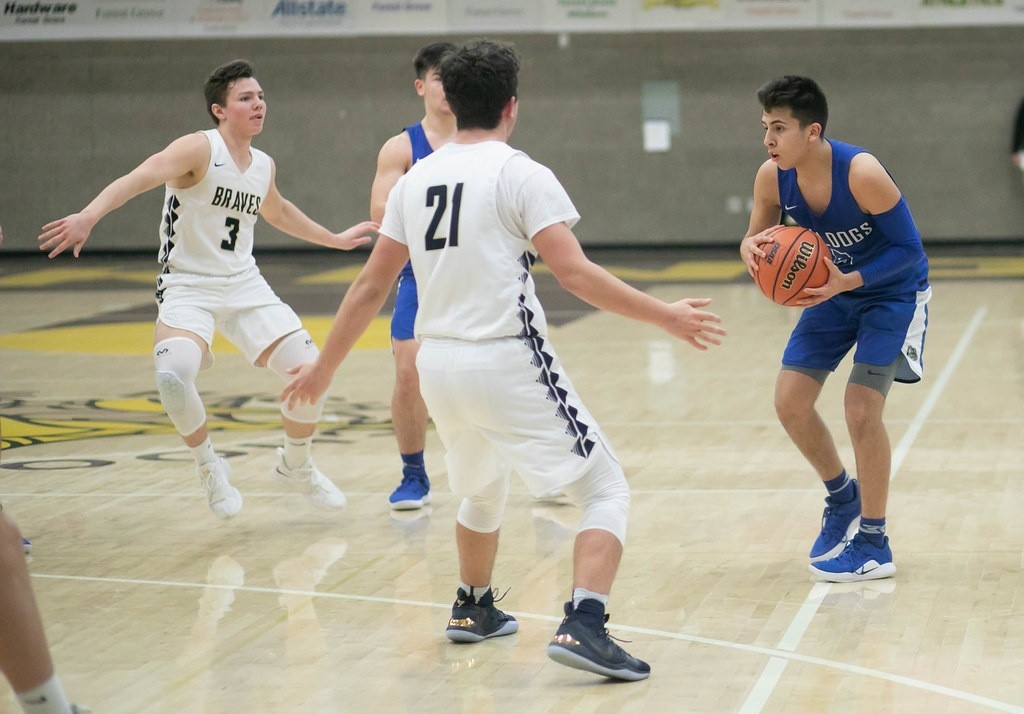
xmin=749 ymin=224 xmax=834 ymax=308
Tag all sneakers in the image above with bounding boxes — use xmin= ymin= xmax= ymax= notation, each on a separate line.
xmin=388 ymin=476 xmax=432 ymax=509
xmin=272 ymin=446 xmax=348 ymax=513
xmin=546 ymin=598 xmax=651 ymax=681
xmin=196 ymin=556 xmax=248 ymax=621
xmin=270 ymin=538 xmax=350 ymax=621
xmin=445 ymin=586 xmax=519 ymax=641
xmin=808 ymin=479 xmax=896 ymax=584
xmin=198 ymin=457 xmax=243 ymax=520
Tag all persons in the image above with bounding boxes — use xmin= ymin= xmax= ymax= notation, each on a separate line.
xmin=739 ymin=75 xmax=932 ymax=584
xmin=1009 ymin=101 xmax=1024 ymax=173
xmin=0 ymin=505 xmax=83 ymax=713
xmin=39 ymin=58 xmax=381 ymax=520
xmin=283 ymin=38 xmax=727 ymax=682
